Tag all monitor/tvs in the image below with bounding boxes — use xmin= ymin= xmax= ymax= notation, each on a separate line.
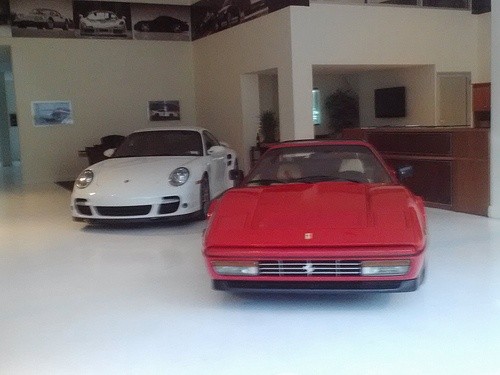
xmin=375 ymin=86 xmax=406 ymax=117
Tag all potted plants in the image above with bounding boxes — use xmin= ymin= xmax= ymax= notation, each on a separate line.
xmin=257 ymin=110 xmax=279 ymax=143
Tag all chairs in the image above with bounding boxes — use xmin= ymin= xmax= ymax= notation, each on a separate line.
xmin=270 ymin=164 xmax=302 ymax=186
xmin=338 ymin=159 xmax=372 ymax=183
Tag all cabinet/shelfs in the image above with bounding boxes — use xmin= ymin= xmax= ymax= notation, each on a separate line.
xmin=343 ymin=82 xmax=491 ymax=216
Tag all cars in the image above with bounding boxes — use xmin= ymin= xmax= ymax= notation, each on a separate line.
xmin=202 ymin=138 xmax=430 ymax=297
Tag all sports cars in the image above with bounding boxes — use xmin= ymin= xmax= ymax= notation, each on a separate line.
xmin=69 ymin=127 xmax=239 ymax=228
